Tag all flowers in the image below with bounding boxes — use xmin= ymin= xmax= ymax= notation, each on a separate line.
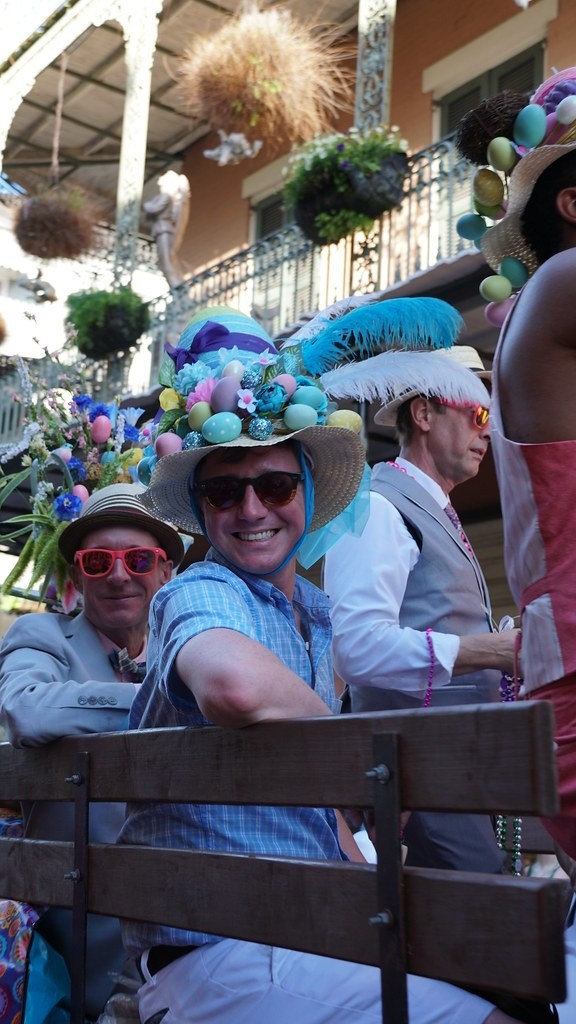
xmin=4 ymin=341 xmax=156 ymax=614
xmin=130 ymin=350 xmax=334 ymax=476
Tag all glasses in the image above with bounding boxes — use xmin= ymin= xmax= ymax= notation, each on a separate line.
xmin=73 ymin=547 xmax=166 ymax=578
xmin=428 ymin=396 xmax=493 ymax=429
xmin=190 ymin=472 xmax=306 ymax=514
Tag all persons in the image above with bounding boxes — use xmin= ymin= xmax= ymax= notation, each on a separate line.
xmin=2 ymin=491 xmax=185 ymax=1024
xmin=143 ymin=170 xmax=190 ymax=290
xmin=477 ymin=63 xmax=576 ymax=864
xmin=119 ymin=397 xmax=532 ymax=1024
xmin=320 ymin=343 xmax=526 ymax=880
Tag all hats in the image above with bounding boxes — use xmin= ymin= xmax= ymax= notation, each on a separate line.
xmin=57 ymin=481 xmax=184 ymax=569
xmin=149 ymin=426 xmax=365 ymax=539
xmin=481 ymin=111 xmax=575 ymax=287
xmin=373 ymin=346 xmax=492 ymax=428
